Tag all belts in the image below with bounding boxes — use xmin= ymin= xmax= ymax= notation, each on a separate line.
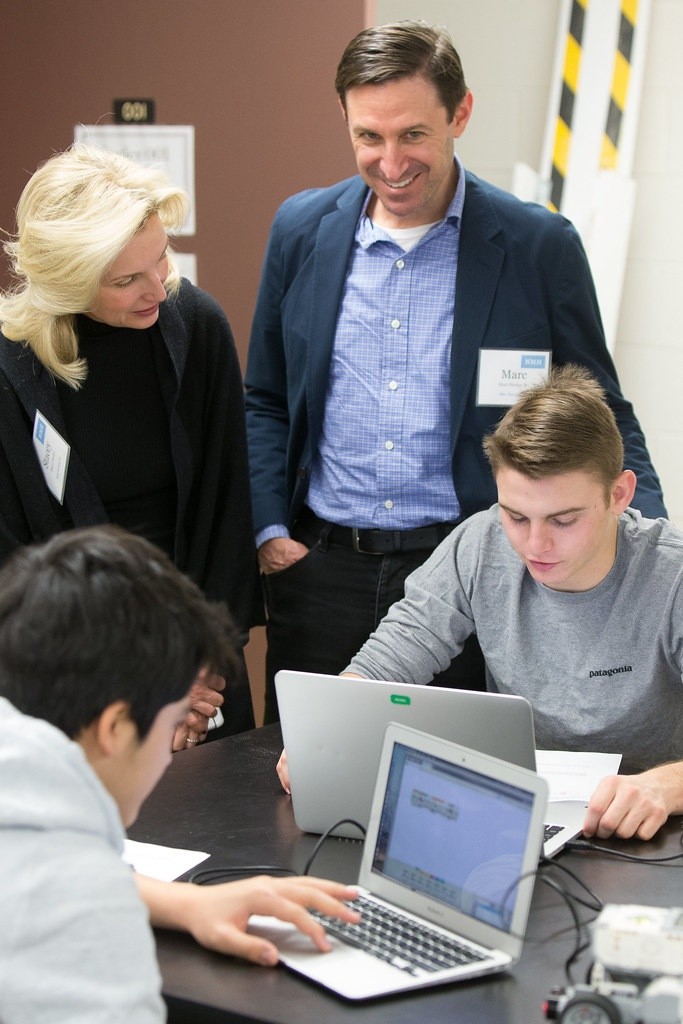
xmin=302 ymin=509 xmax=455 ymax=554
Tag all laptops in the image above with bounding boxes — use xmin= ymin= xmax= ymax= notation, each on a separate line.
xmin=247 ymin=721 xmax=550 ymax=1002
xmin=275 ymin=669 xmax=591 ymax=864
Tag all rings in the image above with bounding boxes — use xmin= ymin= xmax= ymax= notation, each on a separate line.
xmin=187 ymin=738 xmax=198 ymax=743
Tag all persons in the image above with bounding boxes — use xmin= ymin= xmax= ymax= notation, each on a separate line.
xmin=271 ymin=368 xmax=683 ymax=846
xmin=243 ymin=19 xmax=668 ymax=692
xmin=0 ymin=147 xmax=264 ymax=749
xmin=1 ymin=527 xmax=363 ymax=1024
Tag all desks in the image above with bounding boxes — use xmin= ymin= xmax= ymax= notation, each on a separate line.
xmin=123 ymin=721 xmax=682 ymax=1024
